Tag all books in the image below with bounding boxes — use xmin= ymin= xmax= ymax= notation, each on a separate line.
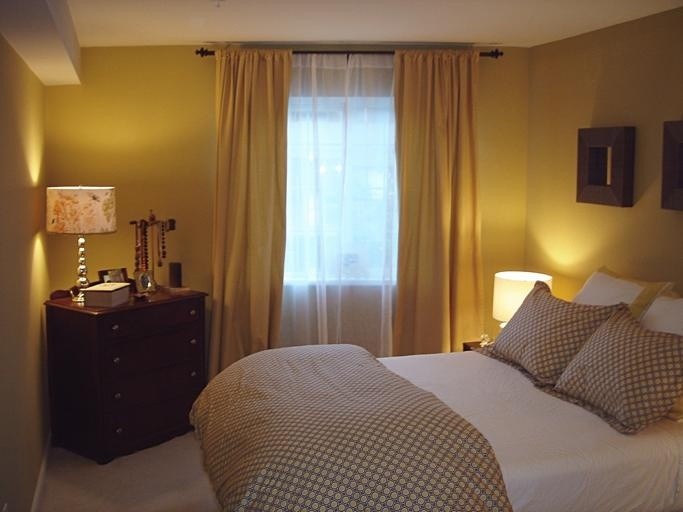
xmin=81 ymin=282 xmax=133 ymax=310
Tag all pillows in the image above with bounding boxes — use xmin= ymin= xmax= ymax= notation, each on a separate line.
xmin=484 ymin=264 xmax=681 ymax=433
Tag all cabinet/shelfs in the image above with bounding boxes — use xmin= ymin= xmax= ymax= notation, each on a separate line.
xmin=46 ymin=285 xmax=211 ymax=468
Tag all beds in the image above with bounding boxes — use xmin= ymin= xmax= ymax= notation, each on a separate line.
xmin=199 ymin=343 xmax=680 ymax=512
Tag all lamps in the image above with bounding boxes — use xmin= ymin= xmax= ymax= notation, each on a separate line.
xmin=45 ymin=183 xmax=118 ymax=302
xmin=489 ymin=268 xmax=555 ymax=331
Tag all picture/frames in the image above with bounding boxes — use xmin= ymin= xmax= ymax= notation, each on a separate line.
xmin=568 ymin=125 xmax=634 ymax=211
xmin=659 ymin=116 xmax=683 ymax=212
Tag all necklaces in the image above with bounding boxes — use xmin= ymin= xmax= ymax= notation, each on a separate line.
xmin=134 ymin=216 xmax=166 ymax=275
